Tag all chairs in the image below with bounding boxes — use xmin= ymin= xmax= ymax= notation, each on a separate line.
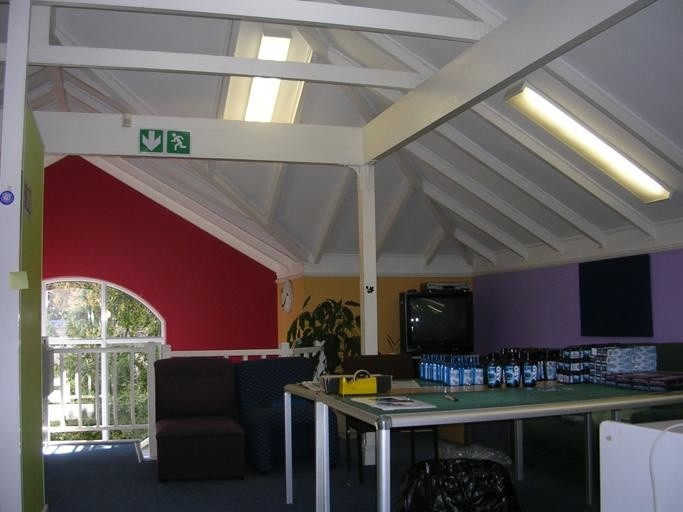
xmin=338 ymin=349 xmax=441 ymax=486
xmin=149 ymin=357 xmax=243 ymax=481
xmin=241 ymin=355 xmax=339 ymax=473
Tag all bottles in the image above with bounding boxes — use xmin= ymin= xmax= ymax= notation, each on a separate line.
xmin=418 ymin=346 xmax=561 ymax=387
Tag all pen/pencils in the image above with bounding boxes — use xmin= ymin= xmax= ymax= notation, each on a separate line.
xmin=445 ymin=394 xmax=458 ymax=401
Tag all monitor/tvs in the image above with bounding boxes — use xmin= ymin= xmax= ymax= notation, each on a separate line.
xmin=399 ymin=290 xmax=474 ymax=355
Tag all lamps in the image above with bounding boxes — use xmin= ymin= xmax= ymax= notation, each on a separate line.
xmin=499 ymin=80 xmax=675 ymax=208
xmin=239 ymin=31 xmax=292 ymax=124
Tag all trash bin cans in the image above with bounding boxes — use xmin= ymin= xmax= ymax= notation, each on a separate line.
xmin=409 ymin=457 xmax=509 ymax=512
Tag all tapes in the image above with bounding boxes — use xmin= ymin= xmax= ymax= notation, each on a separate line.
xmin=353 ymin=369 xmax=371 ymax=381
